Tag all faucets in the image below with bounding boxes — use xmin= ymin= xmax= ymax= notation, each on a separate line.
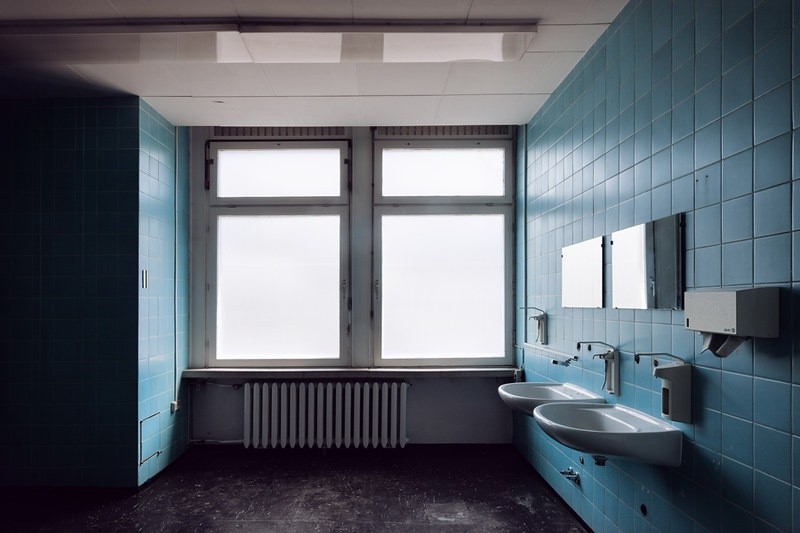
xmin=576 ymin=341 xmax=617 ymax=354
xmin=633 ymin=352 xmax=691 ymax=369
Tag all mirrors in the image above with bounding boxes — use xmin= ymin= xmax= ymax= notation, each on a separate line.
xmin=561 ymin=235 xmax=604 ymax=308
xmin=612 ymin=214 xmax=685 ymax=310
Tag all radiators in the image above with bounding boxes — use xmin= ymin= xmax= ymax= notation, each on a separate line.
xmin=243 ymin=383 xmax=408 ymax=447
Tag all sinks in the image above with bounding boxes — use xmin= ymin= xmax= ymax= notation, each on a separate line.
xmin=497 ymin=381 xmax=605 ymax=418
xmin=532 ymin=402 xmax=683 ymax=468
